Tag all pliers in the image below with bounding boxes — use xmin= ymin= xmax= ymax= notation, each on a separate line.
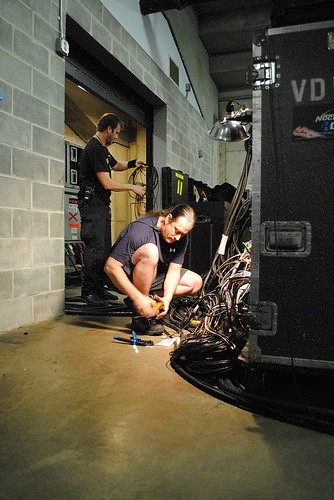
xmin=113 ymin=331 xmax=154 ymax=347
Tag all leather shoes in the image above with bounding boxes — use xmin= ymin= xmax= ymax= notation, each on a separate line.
xmin=81 ymin=293 xmax=107 ymax=307
xmin=99 ymin=291 xmax=118 ymax=301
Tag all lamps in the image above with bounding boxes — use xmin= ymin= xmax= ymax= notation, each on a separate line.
xmin=207 ymin=100 xmax=252 ymax=142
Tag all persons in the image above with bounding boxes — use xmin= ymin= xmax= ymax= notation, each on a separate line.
xmin=102 ymin=201 xmax=204 ymax=337
xmin=77 ymin=112 xmax=147 ymax=308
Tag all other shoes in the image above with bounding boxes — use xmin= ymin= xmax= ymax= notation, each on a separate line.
xmin=130 ymin=317 xmax=164 ymax=336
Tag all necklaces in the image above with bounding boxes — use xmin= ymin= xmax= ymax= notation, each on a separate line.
xmin=94 ymin=133 xmax=102 ymax=145
